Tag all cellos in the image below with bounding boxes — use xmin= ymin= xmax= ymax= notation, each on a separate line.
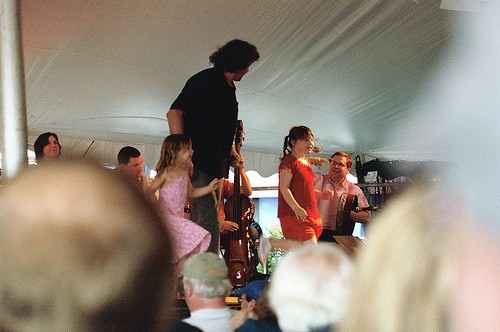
xmin=224 ymin=119 xmax=258 ymax=285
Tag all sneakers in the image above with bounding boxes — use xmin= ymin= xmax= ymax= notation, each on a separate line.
xmin=258 ymin=236 xmax=273 ymax=267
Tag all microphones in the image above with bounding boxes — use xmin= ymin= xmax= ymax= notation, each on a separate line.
xmin=355 ymin=206 xmax=374 ymax=212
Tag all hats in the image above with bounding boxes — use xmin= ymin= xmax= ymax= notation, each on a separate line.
xmin=180 ymin=251 xmax=229 ymax=280
xmin=233 ymin=280 xmax=270 ymax=299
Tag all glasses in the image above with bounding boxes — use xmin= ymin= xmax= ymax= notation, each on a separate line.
xmin=329 ymin=160 xmax=348 ymax=167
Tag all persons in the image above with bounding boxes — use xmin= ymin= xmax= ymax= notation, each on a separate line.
xmin=0 ymin=38 xmax=500 ymax=332
xmin=1 ymin=155 xmax=203 ymax=332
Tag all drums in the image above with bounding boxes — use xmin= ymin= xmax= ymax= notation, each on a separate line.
xmin=335 ymin=191 xmax=359 ymax=237
